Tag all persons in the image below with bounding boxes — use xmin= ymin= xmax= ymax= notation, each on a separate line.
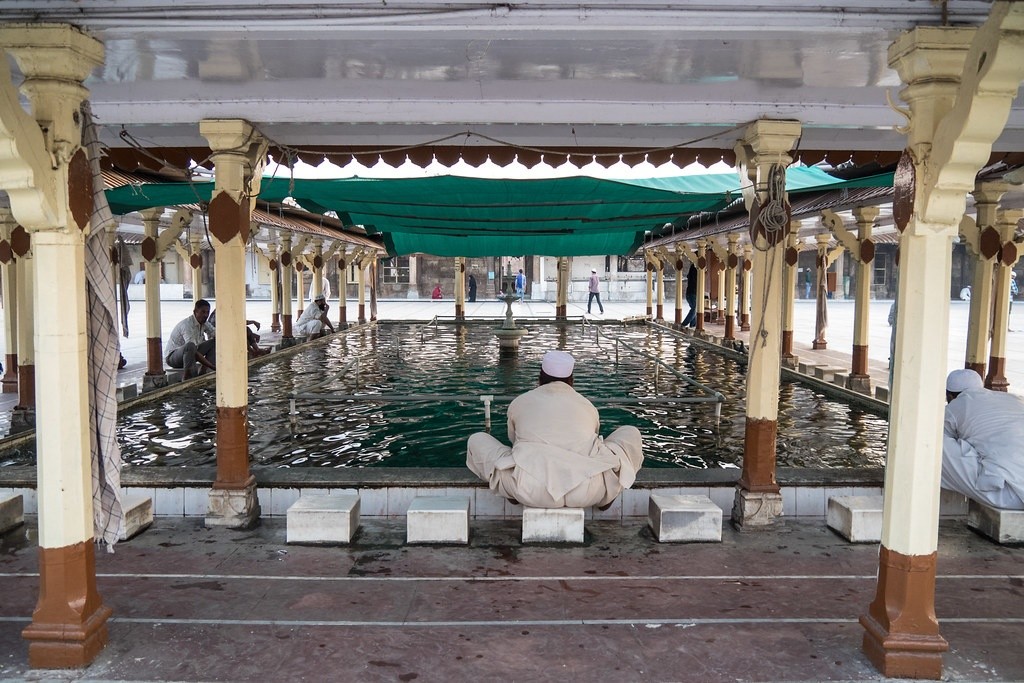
xmin=165 ymin=299 xmax=273 ymax=382
xmin=515 ymin=268 xmax=526 ymax=305
xmin=296 ymin=272 xmax=335 ymax=342
xmin=431 ymin=285 xmax=442 ymax=299
xmin=586 ymin=268 xmax=604 ymax=314
xmin=939 ymin=368 xmax=1024 ymax=510
xmin=466 ymin=350 xmax=644 ymax=511
xmin=680 ymin=263 xmax=1018 ymax=368
xmin=468 ymin=275 xmax=477 ymax=302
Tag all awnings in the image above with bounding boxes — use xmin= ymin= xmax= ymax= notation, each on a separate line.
xmin=103 ymin=170 xmax=912 ymax=259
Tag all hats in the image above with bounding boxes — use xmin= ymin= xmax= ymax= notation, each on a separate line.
xmin=315 ymin=295 xmax=325 ymax=301
xmin=542 ymin=350 xmax=574 ymax=378
xmin=1012 ymin=271 xmax=1017 ymax=277
xmin=967 ymin=285 xmax=972 ymax=288
xmin=946 ymin=369 xmax=984 ymax=392
xmin=592 ymin=268 xmax=596 ymax=273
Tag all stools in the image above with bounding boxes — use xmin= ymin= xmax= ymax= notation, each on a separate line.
xmin=649 ymin=495 xmax=723 ymax=543
xmin=655 ymin=318 xmax=695 ymax=335
xmin=407 ymin=496 xmax=470 ymax=544
xmin=107 ymin=495 xmax=154 ymax=540
xmin=827 ymin=496 xmax=882 ymax=543
xmin=0 ymin=494 xmax=24 ymax=534
xmin=799 ymin=361 xmax=852 ymax=388
xmin=162 ymin=362 xmax=203 ymax=384
xmin=252 ymin=342 xmax=281 ymax=354
xmin=968 ymin=500 xmax=1024 ymax=544
xmin=115 ymin=380 xmax=137 ymax=402
xmin=522 ymin=505 xmax=584 ymax=544
xmin=294 ymin=318 xmax=357 ymax=344
xmin=703 ymin=333 xmax=725 ymax=345
xmin=287 ymin=493 xmax=361 ymax=543
xmin=875 ymin=386 xmax=890 ymax=403
